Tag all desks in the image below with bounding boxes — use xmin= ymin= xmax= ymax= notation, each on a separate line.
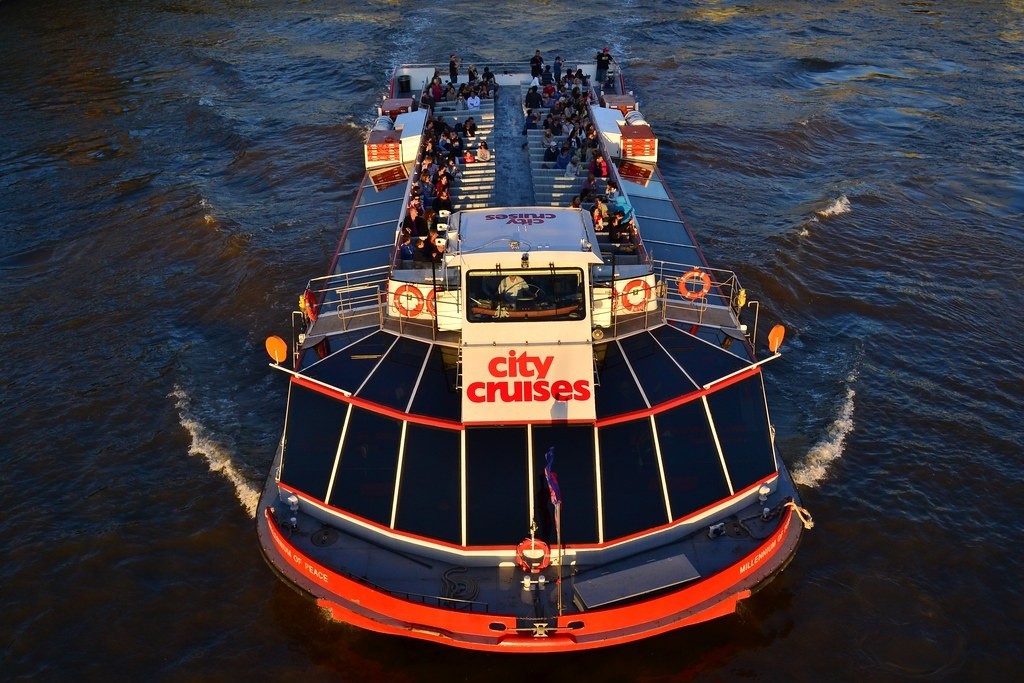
xmin=571 ymin=553 xmax=702 ymax=612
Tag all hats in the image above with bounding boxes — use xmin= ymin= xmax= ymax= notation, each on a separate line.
xmin=603 ymin=48 xmax=609 ymax=52
xmin=572 ymin=155 xmax=581 ymax=161
xmin=550 ymin=141 xmax=557 ymax=146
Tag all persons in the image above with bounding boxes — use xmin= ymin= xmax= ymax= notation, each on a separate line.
xmin=522 ymin=47 xmax=642 ymax=255
xmin=498 ymin=275 xmax=533 ymax=308
xmin=399 ymin=54 xmax=503 ymax=262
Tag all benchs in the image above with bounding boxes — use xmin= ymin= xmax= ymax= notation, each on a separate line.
xmin=395 ymin=78 xmax=639 ymax=270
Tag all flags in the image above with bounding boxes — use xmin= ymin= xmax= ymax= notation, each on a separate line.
xmin=541 ymin=445 xmax=561 ymax=533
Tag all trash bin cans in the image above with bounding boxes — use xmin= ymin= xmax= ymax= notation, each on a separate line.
xmin=398 ymin=75 xmax=410 ymax=92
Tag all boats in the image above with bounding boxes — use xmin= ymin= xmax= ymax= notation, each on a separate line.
xmin=252 ymin=62 xmax=806 ymax=656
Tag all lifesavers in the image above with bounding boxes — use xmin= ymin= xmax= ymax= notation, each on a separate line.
xmin=680 ymin=269 xmax=710 ymax=300
xmin=515 ymin=537 xmax=550 ymax=573
xmin=393 ymin=285 xmax=424 ymax=317
xmin=612 ymin=288 xmax=618 ymax=313
xmin=621 ymin=280 xmax=651 ymax=313
xmin=305 ymin=290 xmax=318 ymax=323
xmin=426 ymin=287 xmax=444 ymax=317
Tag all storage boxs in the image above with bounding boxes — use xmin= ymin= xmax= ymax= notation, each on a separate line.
xmin=367 ymin=130 xmax=403 ymax=161
xmin=367 ymin=161 xmax=409 ymax=193
xmin=381 ymin=97 xmax=415 ymax=119
xmin=618 ymin=124 xmax=656 ymax=156
xmin=600 ymin=94 xmax=635 ymax=117
xmin=618 ymin=160 xmax=655 ymax=187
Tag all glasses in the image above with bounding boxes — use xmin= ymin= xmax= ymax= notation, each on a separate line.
xmin=481 ymin=145 xmax=485 ymax=146
xmin=414 ymin=198 xmax=421 ymax=201
